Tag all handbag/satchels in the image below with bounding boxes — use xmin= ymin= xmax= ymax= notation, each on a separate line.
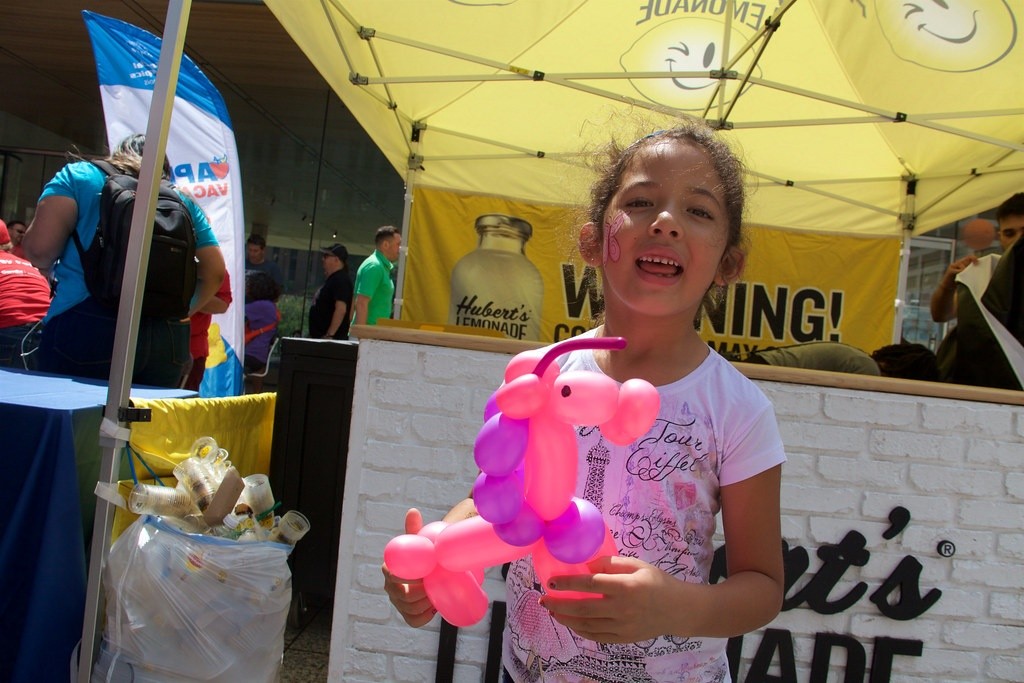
xmin=245 ymin=310 xmax=280 ymax=344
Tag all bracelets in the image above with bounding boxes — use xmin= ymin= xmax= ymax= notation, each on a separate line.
xmin=326 ymin=336 xmax=333 ymax=337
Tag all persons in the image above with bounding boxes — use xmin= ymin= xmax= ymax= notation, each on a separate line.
xmin=0 ymin=134 xmax=232 ymax=392
xmin=244 ymin=272 xmax=281 ymax=373
xmin=348 ymin=225 xmax=400 ymax=341
xmin=742 ymin=341 xmax=935 ymax=380
xmin=930 ymin=192 xmax=1024 ymax=322
xmin=309 ymin=243 xmax=354 ymax=340
xmin=245 ymin=235 xmax=268 ymax=275
xmin=381 ymin=123 xmax=786 ymax=683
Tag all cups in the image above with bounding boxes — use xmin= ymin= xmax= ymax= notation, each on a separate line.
xmin=128 ymin=436 xmax=309 ymax=548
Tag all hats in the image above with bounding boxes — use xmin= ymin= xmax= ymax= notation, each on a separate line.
xmin=321 ymin=244 xmax=347 ymax=263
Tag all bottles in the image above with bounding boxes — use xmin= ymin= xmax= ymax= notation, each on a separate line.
xmin=446 ymin=211 xmax=549 ymax=345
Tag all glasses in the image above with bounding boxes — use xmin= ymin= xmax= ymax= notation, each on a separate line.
xmin=997 ymin=227 xmax=1024 ymax=237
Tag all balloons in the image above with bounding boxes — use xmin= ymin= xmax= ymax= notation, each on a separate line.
xmin=383 ymin=338 xmax=661 ymax=627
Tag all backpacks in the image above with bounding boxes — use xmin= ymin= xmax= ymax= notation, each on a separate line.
xmin=68 ymin=158 xmax=196 ymax=317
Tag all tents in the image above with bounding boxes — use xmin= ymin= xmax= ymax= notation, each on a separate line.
xmin=77 ymin=0 xmax=1024 ymax=683
